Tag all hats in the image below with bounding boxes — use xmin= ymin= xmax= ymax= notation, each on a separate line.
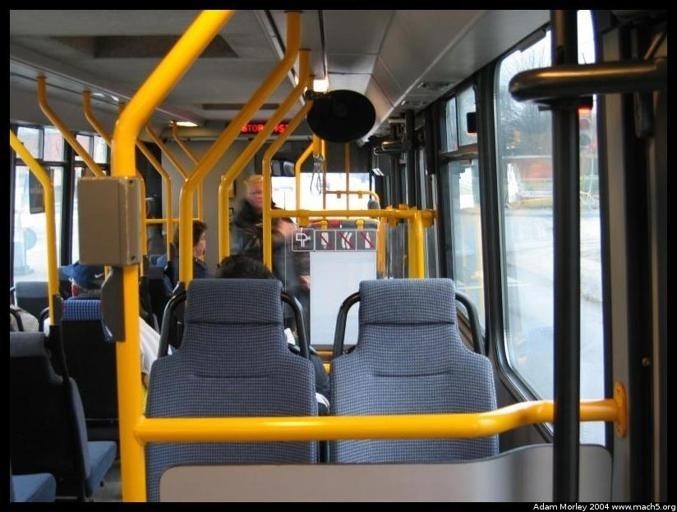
xmin=60 ymin=262 xmax=105 ymax=292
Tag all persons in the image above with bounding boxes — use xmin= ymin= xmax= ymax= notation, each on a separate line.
xmin=368 ymin=201 xmax=381 ymax=219
xmin=42 ymin=172 xmax=331 ymax=400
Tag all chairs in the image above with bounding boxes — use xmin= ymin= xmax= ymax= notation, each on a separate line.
xmin=144 ymin=278 xmax=330 ymax=502
xmin=10 ymin=253 xmax=167 ymax=502
xmin=330 ymin=278 xmax=501 ymax=464
